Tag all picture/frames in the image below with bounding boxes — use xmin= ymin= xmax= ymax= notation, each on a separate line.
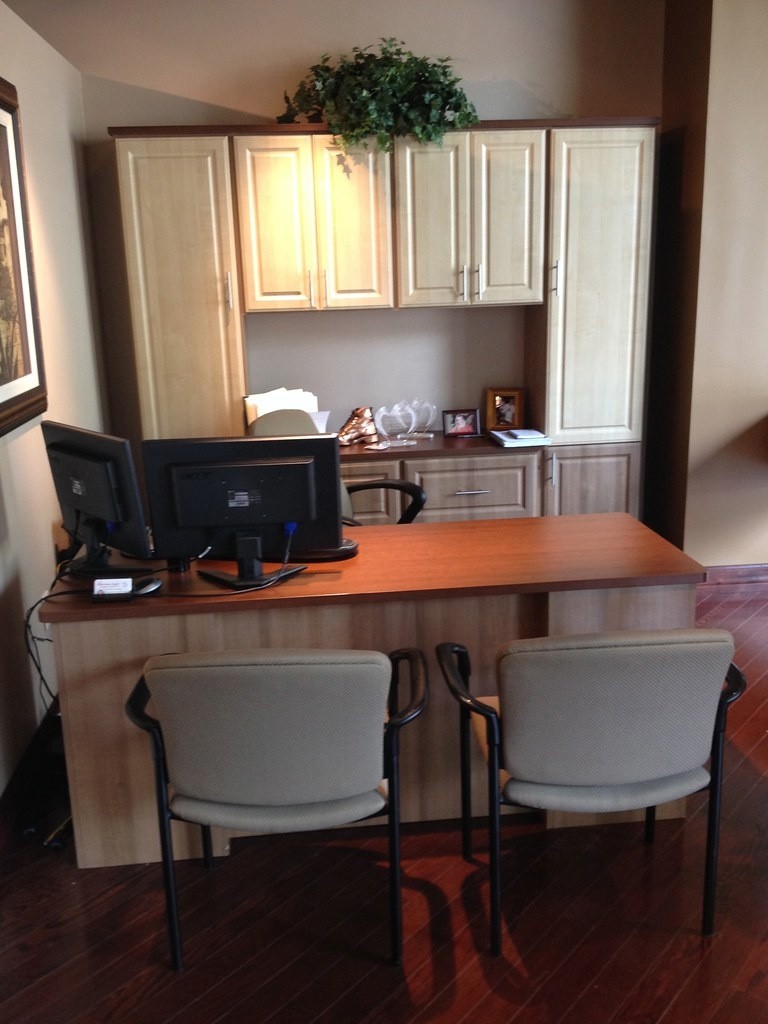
xmin=442 ymin=408 xmax=481 ymax=438
xmin=485 ymin=389 xmax=525 ymax=432
xmin=0 ymin=77 xmax=48 ymax=439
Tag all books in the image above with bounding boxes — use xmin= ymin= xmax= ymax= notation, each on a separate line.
xmin=490 ymin=429 xmax=552 ymax=447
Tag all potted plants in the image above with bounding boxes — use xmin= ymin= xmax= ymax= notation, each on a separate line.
xmin=276 ymin=35 xmax=481 ymax=150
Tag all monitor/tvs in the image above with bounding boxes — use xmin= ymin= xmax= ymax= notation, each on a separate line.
xmin=42 ymin=419 xmax=343 ymax=591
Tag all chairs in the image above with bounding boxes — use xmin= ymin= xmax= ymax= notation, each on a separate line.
xmin=125 ymin=646 xmax=428 ymax=962
xmin=435 ymin=628 xmax=747 ymax=952
xmin=246 ymin=408 xmax=428 ymax=527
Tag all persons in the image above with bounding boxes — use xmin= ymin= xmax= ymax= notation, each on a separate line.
xmin=501 ymin=398 xmax=515 ymax=423
xmin=450 ymin=414 xmax=476 ymax=433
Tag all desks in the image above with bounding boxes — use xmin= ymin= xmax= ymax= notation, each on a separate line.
xmin=38 ymin=512 xmax=706 ymax=868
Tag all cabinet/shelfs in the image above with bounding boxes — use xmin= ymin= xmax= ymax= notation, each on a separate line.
xmin=105 ymin=116 xmax=659 ymax=513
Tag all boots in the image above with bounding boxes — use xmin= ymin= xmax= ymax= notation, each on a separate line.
xmin=338 ymin=407 xmax=378 ymax=445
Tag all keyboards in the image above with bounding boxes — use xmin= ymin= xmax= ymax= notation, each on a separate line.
xmin=260 ymin=538 xmax=358 ymax=563
